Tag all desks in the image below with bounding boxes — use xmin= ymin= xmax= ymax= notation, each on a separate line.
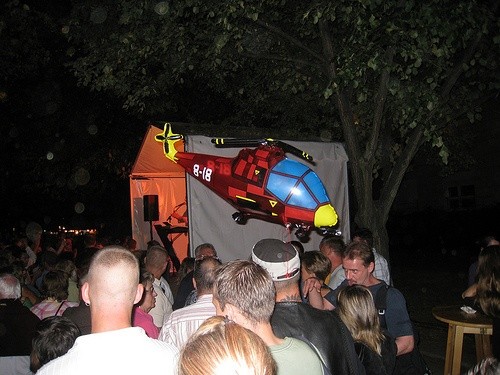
xmin=432 ymin=306 xmax=493 ymax=375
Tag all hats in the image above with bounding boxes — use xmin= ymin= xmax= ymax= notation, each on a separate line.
xmin=250 ymin=238 xmax=301 ymax=282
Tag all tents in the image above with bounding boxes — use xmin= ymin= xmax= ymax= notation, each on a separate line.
xmin=130 ymin=122 xmax=353 ymax=263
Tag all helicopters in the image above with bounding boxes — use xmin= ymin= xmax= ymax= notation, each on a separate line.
xmin=154 ymin=122 xmax=342 ymax=242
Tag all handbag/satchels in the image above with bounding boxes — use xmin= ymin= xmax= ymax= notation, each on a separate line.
xmin=375 ymin=285 xmax=431 ymax=375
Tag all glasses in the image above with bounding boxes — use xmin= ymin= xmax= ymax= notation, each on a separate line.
xmin=196 ymin=255 xmax=219 ymax=261
xmin=142 ymin=286 xmax=155 ymax=293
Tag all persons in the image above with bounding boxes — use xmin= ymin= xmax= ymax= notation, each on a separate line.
xmin=0 ymin=218 xmax=500 ymax=375
